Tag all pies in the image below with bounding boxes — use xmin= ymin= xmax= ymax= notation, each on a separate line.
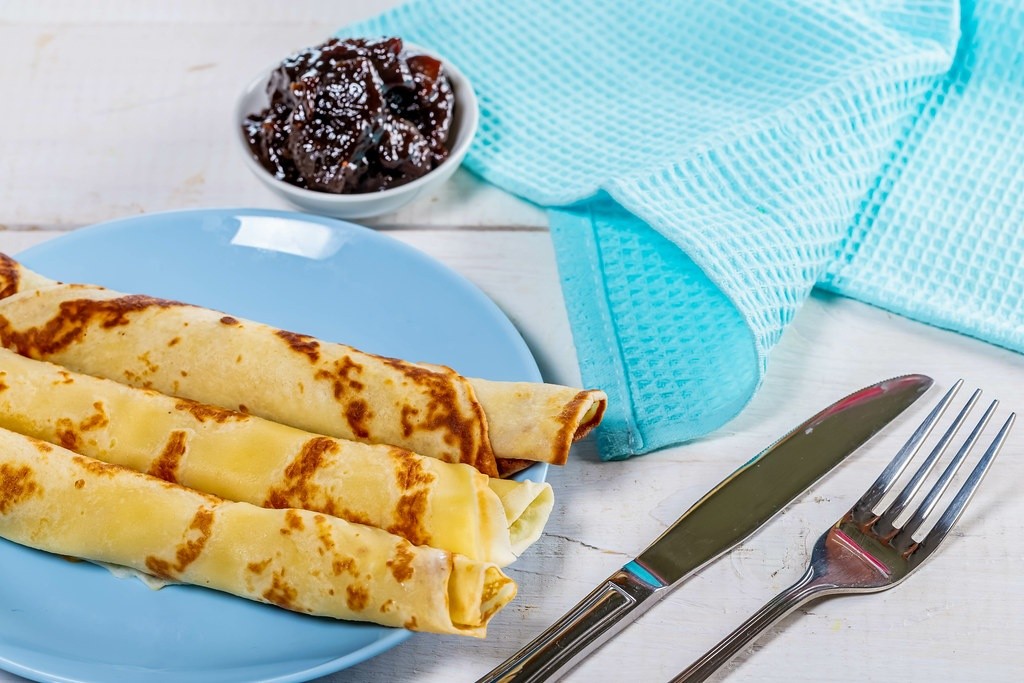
xmin=0 ymin=257 xmax=607 ymax=636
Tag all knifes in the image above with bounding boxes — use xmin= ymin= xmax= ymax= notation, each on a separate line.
xmin=503 ymin=371 xmax=933 ymax=683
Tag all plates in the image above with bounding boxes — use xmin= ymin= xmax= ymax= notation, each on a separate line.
xmin=3 ymin=211 xmax=550 ymax=680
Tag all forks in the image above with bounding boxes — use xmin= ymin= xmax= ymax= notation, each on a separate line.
xmin=669 ymin=376 xmax=1017 ymax=683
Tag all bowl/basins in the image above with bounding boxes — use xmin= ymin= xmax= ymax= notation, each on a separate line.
xmin=229 ymin=40 xmax=479 ymax=218
